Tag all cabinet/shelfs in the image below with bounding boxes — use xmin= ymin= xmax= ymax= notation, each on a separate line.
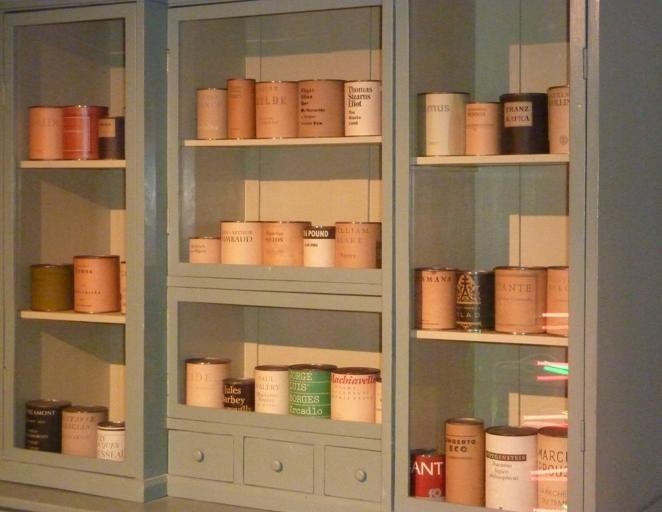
xmin=1 ymin=1 xmax=168 ymax=503
xmin=167 ymin=0 xmax=394 ymax=511
xmin=394 ymin=0 xmax=662 ymax=512
